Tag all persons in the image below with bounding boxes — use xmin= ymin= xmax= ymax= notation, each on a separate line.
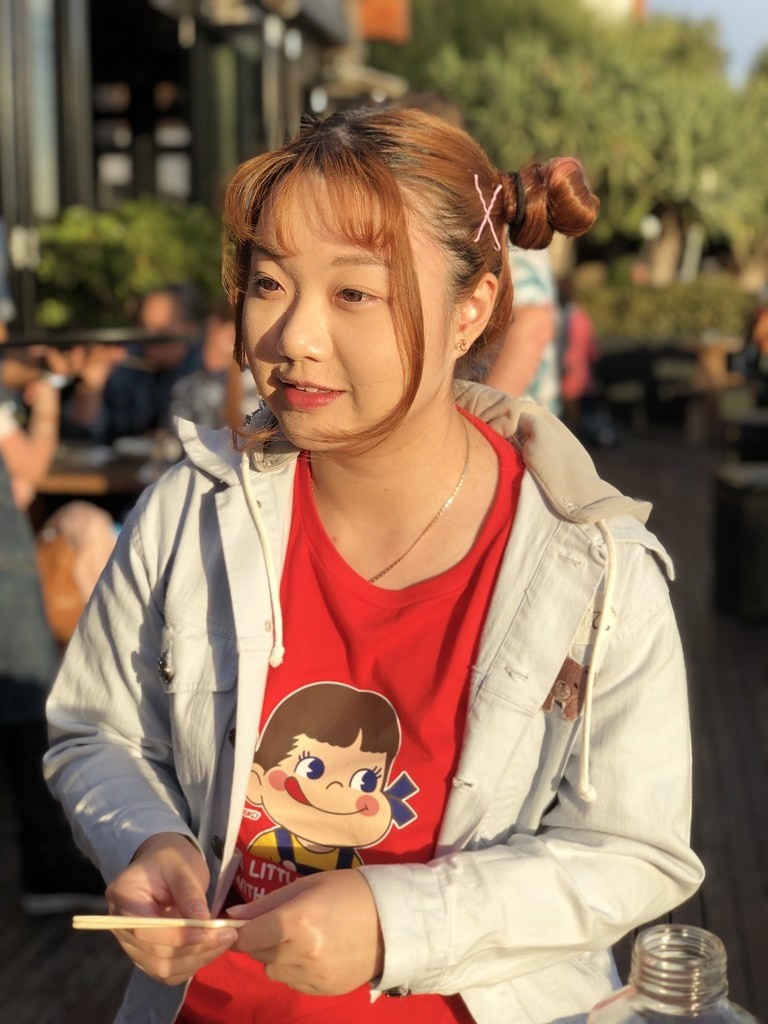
xmin=462 ymin=223 xmax=598 ymax=431
xmin=743 ymin=291 xmax=768 ymax=407
xmin=1 ymin=287 xmax=262 ymax=507
xmin=42 ymin=106 xmax=706 ymax=1024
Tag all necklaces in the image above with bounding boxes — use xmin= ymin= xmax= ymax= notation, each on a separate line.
xmin=310 ymin=413 xmax=471 ymax=583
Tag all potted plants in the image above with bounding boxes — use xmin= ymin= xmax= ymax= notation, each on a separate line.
xmin=27 ymin=195 xmax=228 ymax=331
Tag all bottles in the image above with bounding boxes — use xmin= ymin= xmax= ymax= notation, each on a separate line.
xmin=585 ymin=923 xmax=758 ymax=1023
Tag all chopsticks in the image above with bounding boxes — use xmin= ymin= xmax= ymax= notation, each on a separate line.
xmin=72 ymin=913 xmax=251 ymax=932
